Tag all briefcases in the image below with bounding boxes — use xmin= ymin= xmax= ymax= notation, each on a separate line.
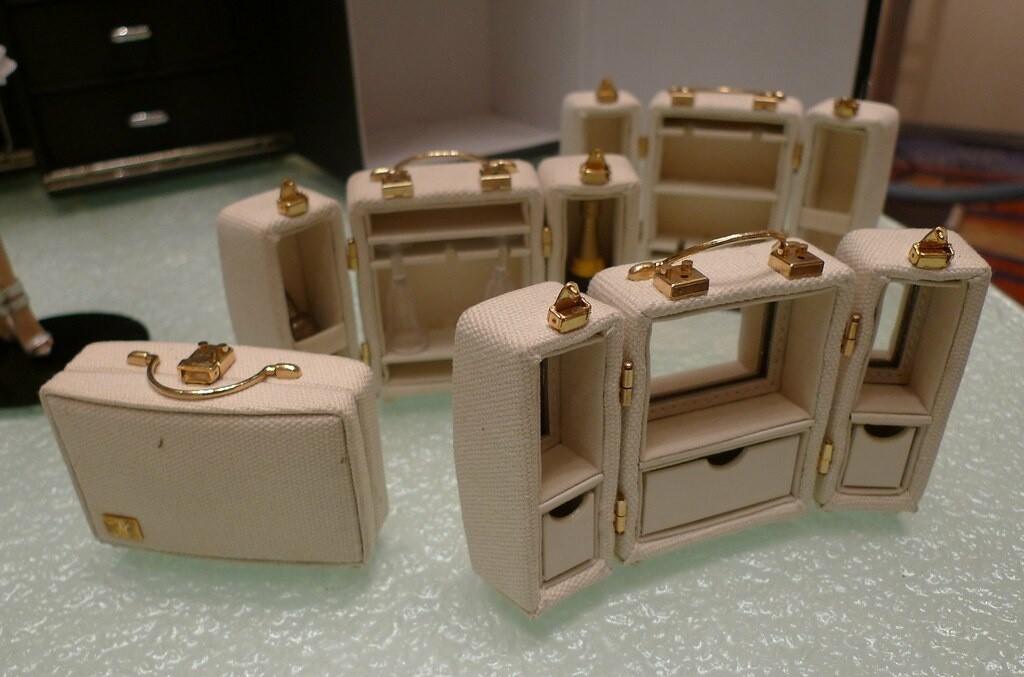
xmin=41 ymin=341 xmax=388 ymax=565
